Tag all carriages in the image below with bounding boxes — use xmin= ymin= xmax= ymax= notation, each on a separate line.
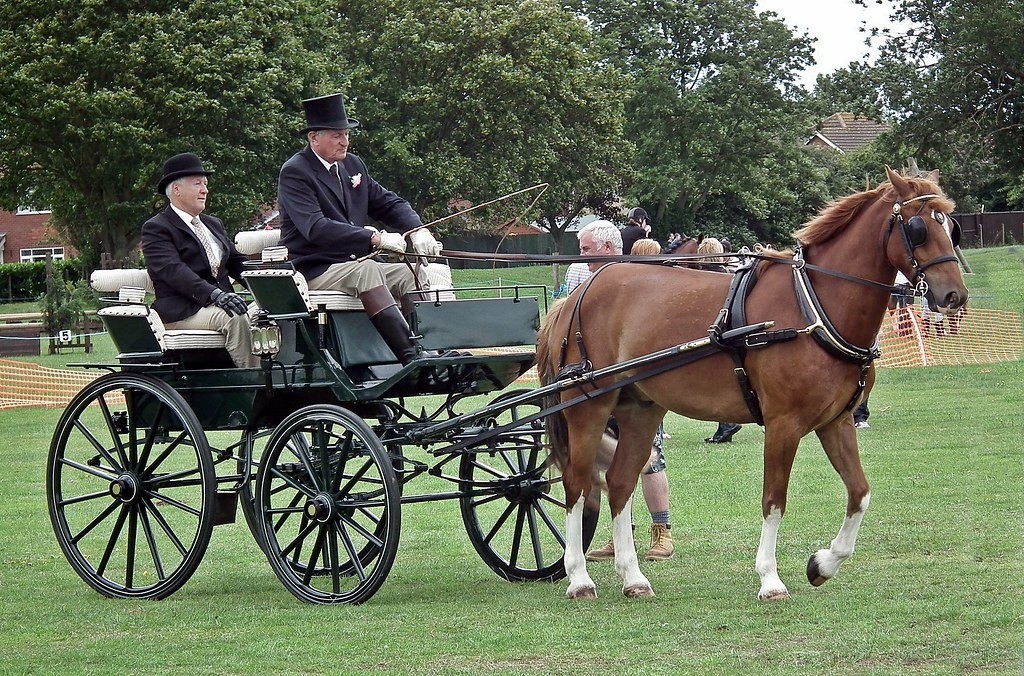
xmin=46 ymin=157 xmax=968 ymax=606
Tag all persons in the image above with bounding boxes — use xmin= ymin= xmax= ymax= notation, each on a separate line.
xmin=563 ymin=206 xmax=871 ymax=443
xmin=277 ymin=92 xmax=440 ymax=368
xmin=140 ymin=152 xmax=259 ymax=368
xmin=577 ymin=219 xmax=676 ymax=561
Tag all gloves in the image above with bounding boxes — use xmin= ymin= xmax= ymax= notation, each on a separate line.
xmin=210 ymin=288 xmax=248 ymax=317
xmin=410 ymin=228 xmax=440 ymax=267
xmin=376 ymin=229 xmax=407 ymax=257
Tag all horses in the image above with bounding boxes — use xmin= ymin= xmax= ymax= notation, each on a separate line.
xmin=535 ymin=164 xmax=968 ymax=596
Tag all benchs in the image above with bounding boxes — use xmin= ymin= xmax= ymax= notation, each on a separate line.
xmin=89 ymin=269 xmax=251 ymax=358
xmin=234 ymin=229 xmax=455 ymax=317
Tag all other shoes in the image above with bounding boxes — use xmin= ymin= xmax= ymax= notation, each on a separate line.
xmin=662 ymin=431 xmax=671 ymax=440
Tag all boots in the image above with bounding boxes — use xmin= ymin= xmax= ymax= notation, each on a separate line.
xmin=369 ymin=303 xmax=459 ymax=383
xmin=405 ymin=309 xmax=473 ymax=385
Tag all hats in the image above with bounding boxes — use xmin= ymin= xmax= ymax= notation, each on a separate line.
xmin=628 ymin=207 xmax=650 ymax=223
xmin=720 ymin=239 xmax=730 ymax=252
xmin=297 ymin=93 xmax=360 ymax=135
xmin=158 ymin=152 xmax=215 ymax=195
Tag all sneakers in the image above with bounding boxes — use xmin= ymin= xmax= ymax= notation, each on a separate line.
xmin=588 ymin=524 xmax=637 ymax=561
xmin=705 ymin=422 xmax=742 ymax=444
xmin=855 ymin=421 xmax=871 ymax=429
xmin=645 ymin=522 xmax=675 ymax=560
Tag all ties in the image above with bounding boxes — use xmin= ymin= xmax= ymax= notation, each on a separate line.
xmin=329 ymin=165 xmax=343 ymax=196
xmin=191 ymin=218 xmax=220 ymax=278
